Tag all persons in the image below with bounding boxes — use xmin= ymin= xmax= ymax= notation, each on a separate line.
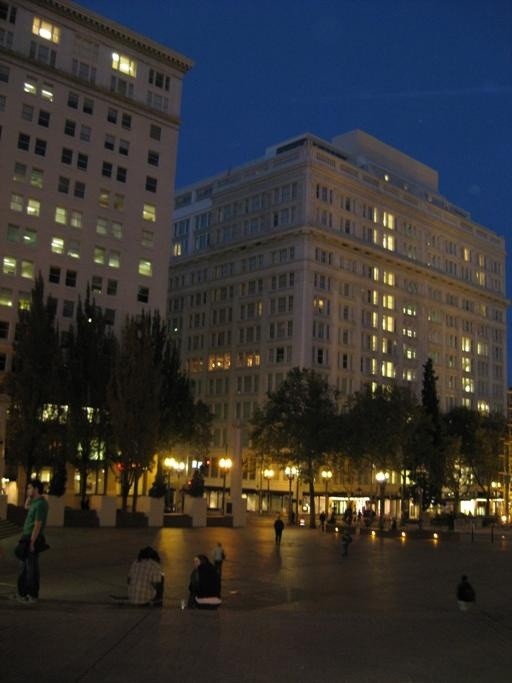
xmin=340 ymin=529 xmax=352 ymax=557
xmin=125 ymin=543 xmax=165 ymax=609
xmin=8 ymin=478 xmax=50 ymax=605
xmin=288 ymin=509 xmax=295 ymax=526
xmin=273 ymin=514 xmax=285 ymax=544
xmin=186 ymin=551 xmax=221 ymax=613
xmin=455 ymin=573 xmax=476 ymax=612
xmin=210 ymin=540 xmax=225 ymax=579
xmin=317 ymin=505 xmax=378 ymax=533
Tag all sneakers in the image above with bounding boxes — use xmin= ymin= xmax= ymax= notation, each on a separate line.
xmin=16 ymin=594 xmax=40 ymax=605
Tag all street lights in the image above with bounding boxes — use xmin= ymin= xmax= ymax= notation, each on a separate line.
xmin=376 ymin=471 xmax=390 ymax=530
xmin=492 ymin=480 xmax=501 ymax=515
xmin=322 ymin=470 xmax=332 ymax=519
xmin=285 ymin=465 xmax=297 ymax=513
xmin=263 ymin=468 xmax=274 ymax=513
xmin=219 ymin=457 xmax=232 ymax=515
xmin=164 ymin=456 xmax=185 ymax=512
